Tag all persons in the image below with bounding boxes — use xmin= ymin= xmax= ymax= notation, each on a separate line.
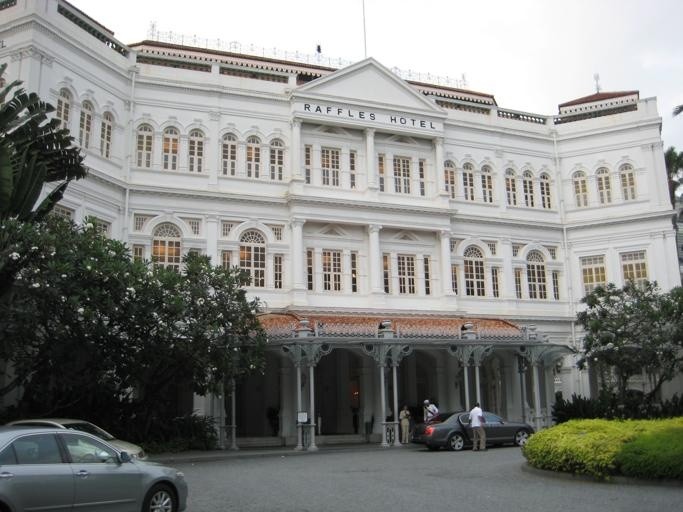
xmin=468 ymin=403 xmax=487 ymax=451
xmin=421 ymin=400 xmax=438 ymax=424
xmin=398 ymin=404 xmax=412 ymax=444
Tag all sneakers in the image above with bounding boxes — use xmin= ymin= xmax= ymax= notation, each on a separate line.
xmin=473 ymin=447 xmax=488 ymax=451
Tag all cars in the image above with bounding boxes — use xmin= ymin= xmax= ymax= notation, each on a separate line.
xmin=409 ymin=411 xmax=535 ymax=451
xmin=0 ymin=418 xmax=189 ymax=512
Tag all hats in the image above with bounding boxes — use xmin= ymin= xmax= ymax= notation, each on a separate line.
xmin=424 ymin=400 xmax=429 ymax=404
